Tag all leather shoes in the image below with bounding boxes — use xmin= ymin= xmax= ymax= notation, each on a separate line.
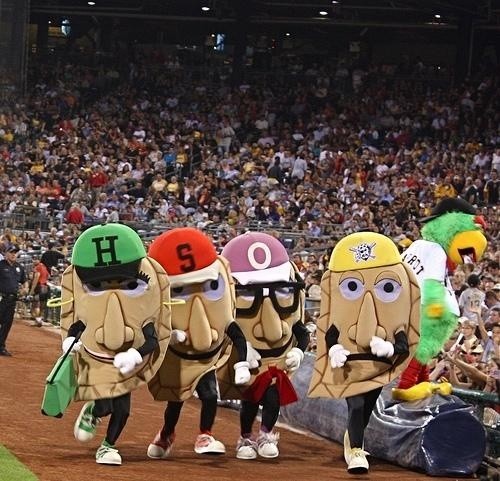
xmin=1 ymin=349 xmax=10 ymax=355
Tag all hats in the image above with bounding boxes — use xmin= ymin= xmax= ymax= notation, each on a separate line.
xmin=6 ymin=243 xmax=20 ymax=252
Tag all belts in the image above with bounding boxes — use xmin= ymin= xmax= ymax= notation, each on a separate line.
xmin=0 ymin=292 xmax=15 ymax=297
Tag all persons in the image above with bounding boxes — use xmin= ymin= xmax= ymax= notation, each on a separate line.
xmin=139 ymin=224 xmax=251 ymax=462
xmin=217 ymin=229 xmax=312 ymax=459
xmin=0 ymin=32 xmax=499 ymax=262
xmin=308 ymin=228 xmax=423 ymax=474
xmin=41 ymin=220 xmax=167 ymax=468
xmin=286 ymin=254 xmax=330 ymax=356
xmin=0 ymin=244 xmax=26 ymax=357
xmin=426 ymin=256 xmax=500 ymax=396
xmin=9 ymin=244 xmax=72 ymax=329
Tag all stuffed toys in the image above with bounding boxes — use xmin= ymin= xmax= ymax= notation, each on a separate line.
xmin=388 ymin=195 xmax=487 ymax=398
